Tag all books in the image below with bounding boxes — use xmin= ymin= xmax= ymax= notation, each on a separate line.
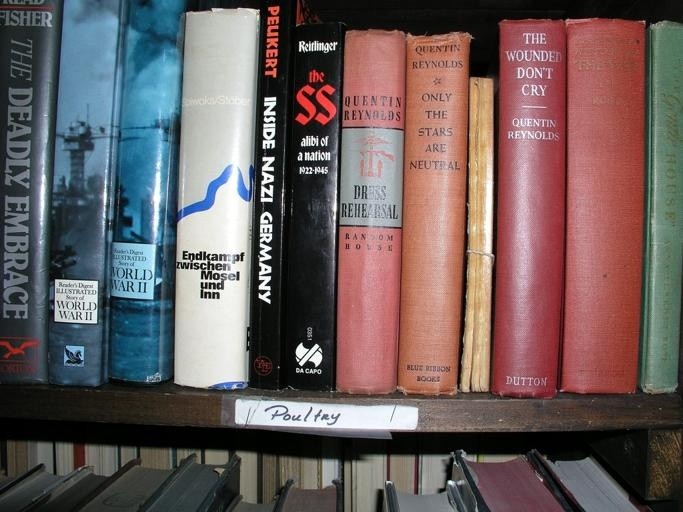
xmin=0 ymin=450 xmax=641 ymax=511
xmin=0 ymin=1 xmax=682 ymax=399
